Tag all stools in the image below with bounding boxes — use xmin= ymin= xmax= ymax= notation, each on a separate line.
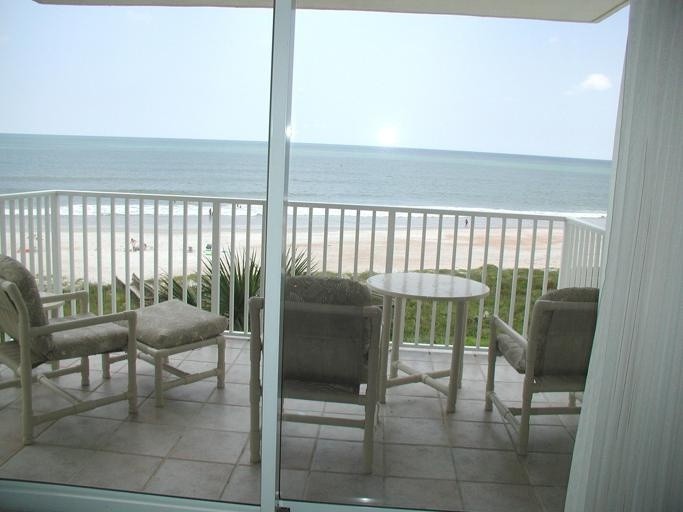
xmin=122 ymin=295 xmax=228 ymax=407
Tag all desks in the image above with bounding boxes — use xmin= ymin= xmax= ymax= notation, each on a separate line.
xmin=361 ymin=271 xmax=489 ymax=415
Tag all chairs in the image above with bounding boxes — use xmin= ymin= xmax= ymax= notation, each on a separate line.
xmin=0 ymin=255 xmax=140 ymax=445
xmin=482 ymin=287 xmax=601 ymax=457
xmin=242 ymin=273 xmax=383 ymax=478
